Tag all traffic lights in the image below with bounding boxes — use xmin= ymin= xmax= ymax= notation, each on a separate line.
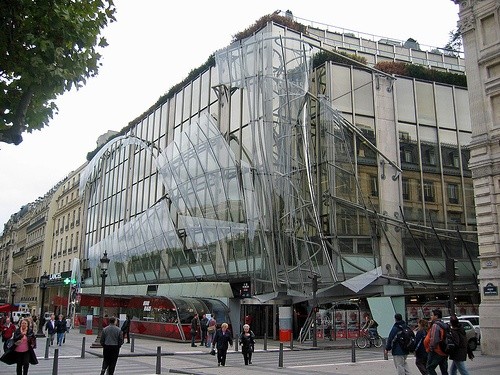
xmin=314 ymin=307 xmax=319 ymax=312
xmin=445 ymin=257 xmax=460 ymax=281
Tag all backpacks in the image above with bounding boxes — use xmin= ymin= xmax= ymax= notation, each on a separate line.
xmin=373 ymin=319 xmax=378 ymax=326
xmin=397 ymin=322 xmax=416 ymax=354
xmin=436 ymin=321 xmax=459 ymax=355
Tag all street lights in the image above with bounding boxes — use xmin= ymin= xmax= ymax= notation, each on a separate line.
xmin=10 ymin=283 xmax=17 ymax=323
xmin=89 ymin=250 xmax=111 ymax=349
xmin=34 ymin=269 xmax=48 ymax=338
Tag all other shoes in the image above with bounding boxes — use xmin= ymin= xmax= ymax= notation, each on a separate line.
xmin=249 ymin=361 xmax=252 ymax=364
xmin=100 ymin=369 xmax=105 ymax=375
xmin=218 ymin=364 xmax=220 ymax=366
xmin=245 ymin=361 xmax=248 ymax=365
xmin=370 ymin=338 xmax=374 ymax=339
xmin=192 ymin=345 xmax=197 ymax=347
xmin=200 ymin=345 xmax=203 ymax=346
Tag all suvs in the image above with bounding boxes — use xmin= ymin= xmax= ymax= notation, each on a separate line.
xmin=413 ymin=315 xmax=480 ymax=350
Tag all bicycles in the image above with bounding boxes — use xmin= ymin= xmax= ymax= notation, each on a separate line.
xmin=356 ymin=331 xmax=383 ymax=348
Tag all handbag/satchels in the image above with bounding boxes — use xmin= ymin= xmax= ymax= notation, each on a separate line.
xmin=63 ymin=337 xmax=65 ymax=343
xmin=6 ymin=341 xmax=15 ymax=350
xmin=207 ymin=326 xmax=214 ymax=334
xmin=191 ymin=328 xmax=194 ymax=333
xmin=211 ymin=349 xmax=215 ymax=355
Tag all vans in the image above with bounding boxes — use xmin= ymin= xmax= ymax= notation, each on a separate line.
xmin=10 ymin=311 xmax=33 ymax=325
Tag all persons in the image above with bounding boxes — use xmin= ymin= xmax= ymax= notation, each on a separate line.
xmin=0 ymin=310 xmax=475 ymax=375
xmin=0 ymin=319 xmax=39 ymax=375
xmin=212 ymin=322 xmax=233 ymax=366
xmin=100 ymin=316 xmax=124 ymax=375
xmin=238 ymin=324 xmax=255 ymax=365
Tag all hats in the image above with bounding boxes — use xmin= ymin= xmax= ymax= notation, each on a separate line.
xmin=210 ymin=313 xmax=214 ymax=316
xmin=59 ymin=314 xmax=63 ymax=317
